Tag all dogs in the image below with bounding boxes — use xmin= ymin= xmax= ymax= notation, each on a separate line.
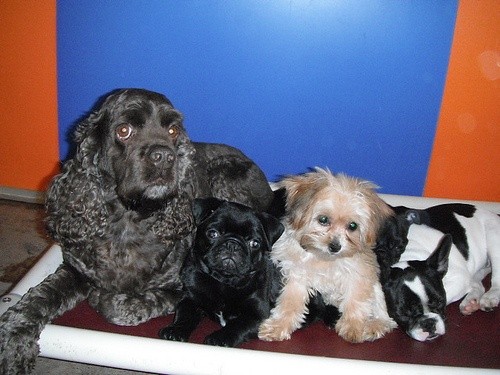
xmin=258 ymin=166 xmax=400 ymax=344
xmin=0 ymin=88 xmax=274 ymax=375
xmin=372 ymin=203 xmax=500 ymax=343
xmin=159 ymin=196 xmax=340 ymax=349
